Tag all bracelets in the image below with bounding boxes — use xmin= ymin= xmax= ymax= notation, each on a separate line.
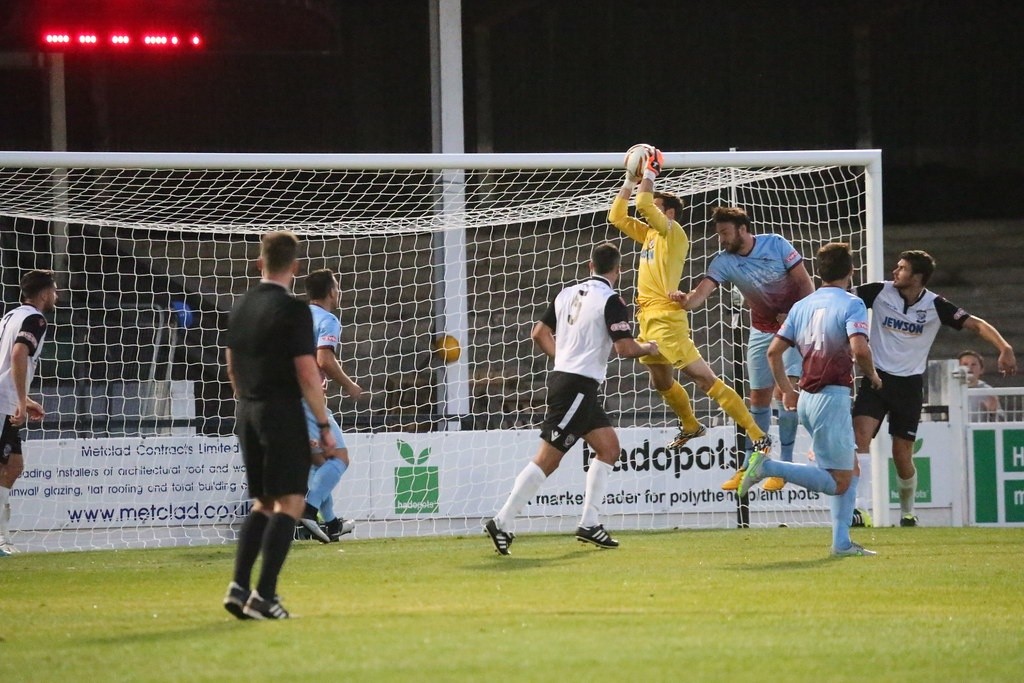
xmin=317 ymin=422 xmax=330 ymax=428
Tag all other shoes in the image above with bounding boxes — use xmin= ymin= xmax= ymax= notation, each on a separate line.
xmin=0 ymin=533 xmax=21 ymax=556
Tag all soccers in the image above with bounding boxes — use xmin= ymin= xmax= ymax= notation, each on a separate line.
xmin=624 ymin=143 xmax=655 ymax=180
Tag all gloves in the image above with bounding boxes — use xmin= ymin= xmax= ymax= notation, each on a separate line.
xmin=641 ymin=147 xmax=664 ymax=182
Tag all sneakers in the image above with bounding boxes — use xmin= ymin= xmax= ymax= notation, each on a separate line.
xmin=737 ymin=452 xmax=771 ymax=499
xmin=753 ymin=434 xmax=778 ymax=458
xmin=328 ymin=517 xmax=355 ymax=542
xmin=223 ymin=581 xmax=251 ymax=620
xmin=481 ymin=517 xmax=515 ymax=556
xmin=900 ymin=514 xmax=919 ymax=529
xmin=666 ymin=424 xmax=706 ymax=449
xmin=576 ymin=523 xmax=619 ymax=549
xmin=764 ymin=477 xmax=786 ymax=492
xmin=299 ymin=516 xmax=331 ymax=545
xmin=722 ymin=468 xmax=745 ymax=491
xmin=850 ymin=507 xmax=873 ymax=529
xmin=830 ymin=541 xmax=879 ymax=559
xmin=243 ymin=590 xmax=303 ymax=620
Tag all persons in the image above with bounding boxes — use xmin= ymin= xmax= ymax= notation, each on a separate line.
xmin=845 ymin=249 xmax=1018 ymax=527
xmin=292 ymin=269 xmax=362 ymax=544
xmin=0 ymin=270 xmax=59 ymax=557
xmin=224 ymin=231 xmax=337 ymax=620
xmin=485 ymin=244 xmax=658 ymax=556
xmin=736 ymin=244 xmax=878 ymax=558
xmin=608 ymin=147 xmax=772 ymax=457
xmin=959 ymin=349 xmax=1006 ymax=422
xmin=668 ymin=206 xmax=815 ymax=491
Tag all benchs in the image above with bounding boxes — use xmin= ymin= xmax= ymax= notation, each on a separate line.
xmin=135 ymin=221 xmax=1024 ymax=430
